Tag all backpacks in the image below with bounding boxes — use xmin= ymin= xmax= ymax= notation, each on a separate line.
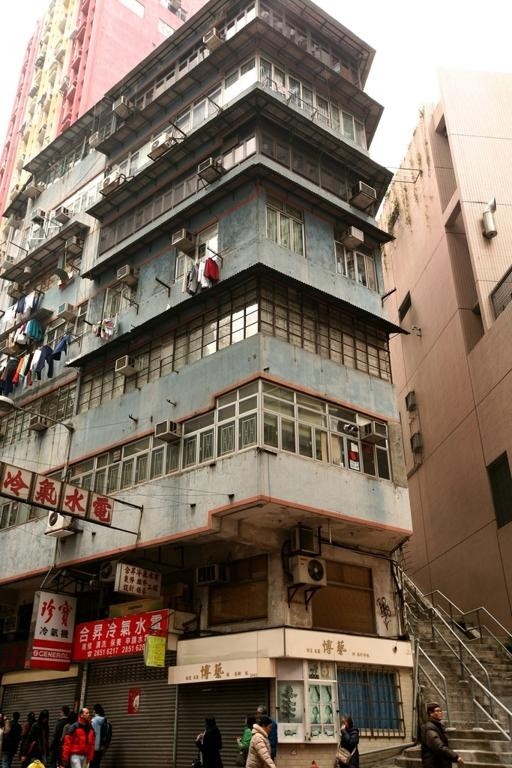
xmin=100 ymin=718 xmax=112 ymax=746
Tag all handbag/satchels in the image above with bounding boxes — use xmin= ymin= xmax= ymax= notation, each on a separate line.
xmin=191 ymin=759 xmax=201 ymax=768
xmin=236 ymin=750 xmax=247 ymax=766
xmin=335 ymin=747 xmax=351 ymax=764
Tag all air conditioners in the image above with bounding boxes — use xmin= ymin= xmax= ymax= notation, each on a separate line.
xmin=28 ymin=414 xmax=50 ymax=432
xmin=195 ymin=563 xmax=231 ymax=588
xmin=339 ymin=179 xmax=377 ymax=251
xmin=44 ymin=510 xmax=84 ymax=540
xmin=0 ymin=208 xmax=76 ymax=356
xmin=114 ymin=354 xmax=138 ymax=377
xmin=154 ymin=420 xmax=182 ymax=444
xmin=99 ymin=559 xmax=121 ymax=582
xmin=360 ymin=421 xmax=389 ymax=444
xmin=287 ymin=524 xmax=328 ymax=587
xmin=90 ymin=96 xmax=226 ymax=288
xmin=203 ymin=27 xmax=225 ymax=53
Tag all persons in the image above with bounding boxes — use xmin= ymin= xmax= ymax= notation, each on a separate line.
xmin=194 ymin=715 xmax=226 ymax=767
xmin=244 ymin=715 xmax=278 ymax=768
xmin=335 ymin=713 xmax=361 ymax=768
xmin=0 ymin=702 xmax=113 ymax=768
xmin=256 ymin=705 xmax=278 ymax=762
xmin=420 ymin=702 xmax=469 ymax=768
xmin=236 ymin=714 xmax=256 ymax=768
